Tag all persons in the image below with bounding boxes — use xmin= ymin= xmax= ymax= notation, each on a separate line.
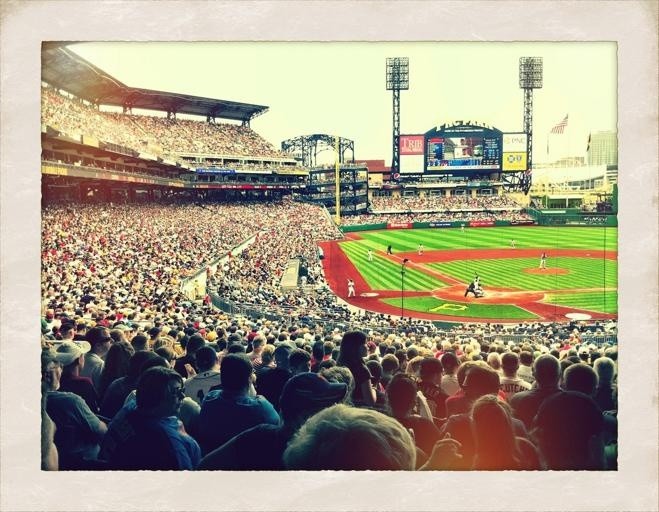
xmin=40 ymin=90 xmax=618 ymax=472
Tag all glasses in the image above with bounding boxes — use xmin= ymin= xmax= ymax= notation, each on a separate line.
xmin=173 ymin=387 xmax=186 ymax=394
xmin=102 ymin=337 xmax=111 ymax=342
xmin=459 ymin=384 xmax=469 ymax=392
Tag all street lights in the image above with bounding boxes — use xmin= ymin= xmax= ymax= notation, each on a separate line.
xmin=386 ymin=57 xmax=409 ymax=183
xmin=400 ymin=258 xmax=409 ymax=319
xmin=519 ymin=56 xmax=543 ymax=196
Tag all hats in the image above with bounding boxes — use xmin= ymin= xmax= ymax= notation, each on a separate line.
xmin=280 ymin=373 xmax=348 ymax=411
xmin=60 ymin=322 xmax=75 ymax=331
xmin=57 ymin=340 xmax=92 ymax=365
xmin=47 ymin=343 xmax=74 ymax=362
xmin=42 ymin=328 xmax=51 ymax=334
xmin=151 ymin=327 xmax=160 ymax=336
